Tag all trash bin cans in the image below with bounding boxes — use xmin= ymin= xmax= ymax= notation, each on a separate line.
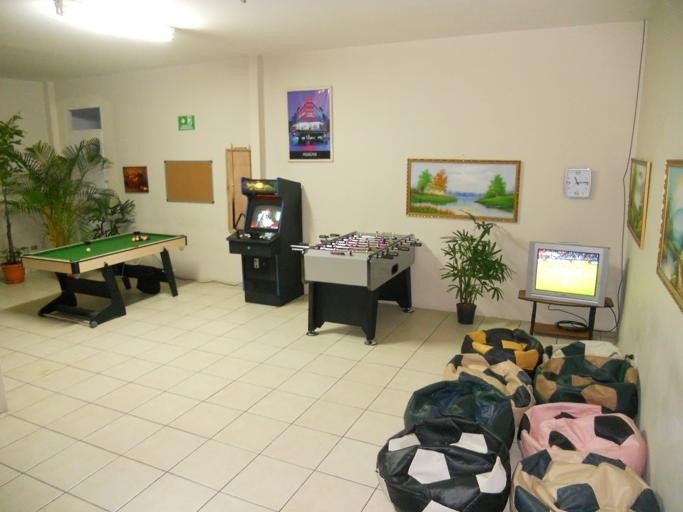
xmin=136 ymin=265 xmax=162 ymax=294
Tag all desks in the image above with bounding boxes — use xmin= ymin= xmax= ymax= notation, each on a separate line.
xmin=23 ymin=231 xmax=187 ymax=326
xmin=517 ymin=289 xmax=614 ymax=341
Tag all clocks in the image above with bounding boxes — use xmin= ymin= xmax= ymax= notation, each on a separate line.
xmin=564 ymin=168 xmax=593 ymax=199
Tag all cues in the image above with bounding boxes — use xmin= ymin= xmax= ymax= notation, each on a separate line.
xmin=230 ymin=144 xmax=236 ymax=235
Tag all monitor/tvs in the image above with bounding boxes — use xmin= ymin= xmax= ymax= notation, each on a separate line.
xmin=525 ymin=241 xmax=610 ymax=308
xmin=249 ymin=204 xmax=282 ymax=230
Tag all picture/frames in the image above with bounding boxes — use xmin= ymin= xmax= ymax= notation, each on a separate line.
xmin=122 ymin=166 xmax=149 ymax=193
xmin=407 ymin=158 xmax=521 ymax=221
xmin=286 ymin=87 xmax=332 ymax=162
xmin=626 ymin=155 xmax=651 ymax=250
xmin=654 ymin=158 xmax=683 ymax=313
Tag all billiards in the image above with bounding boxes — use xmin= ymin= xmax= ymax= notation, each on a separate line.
xmin=131 ymin=234 xmax=149 ymax=243
xmin=86 ymin=247 xmax=91 ymax=252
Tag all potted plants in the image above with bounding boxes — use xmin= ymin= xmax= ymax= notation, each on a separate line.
xmin=440 ymin=210 xmax=512 ymax=324
xmin=0 ymin=108 xmax=29 ymax=284
